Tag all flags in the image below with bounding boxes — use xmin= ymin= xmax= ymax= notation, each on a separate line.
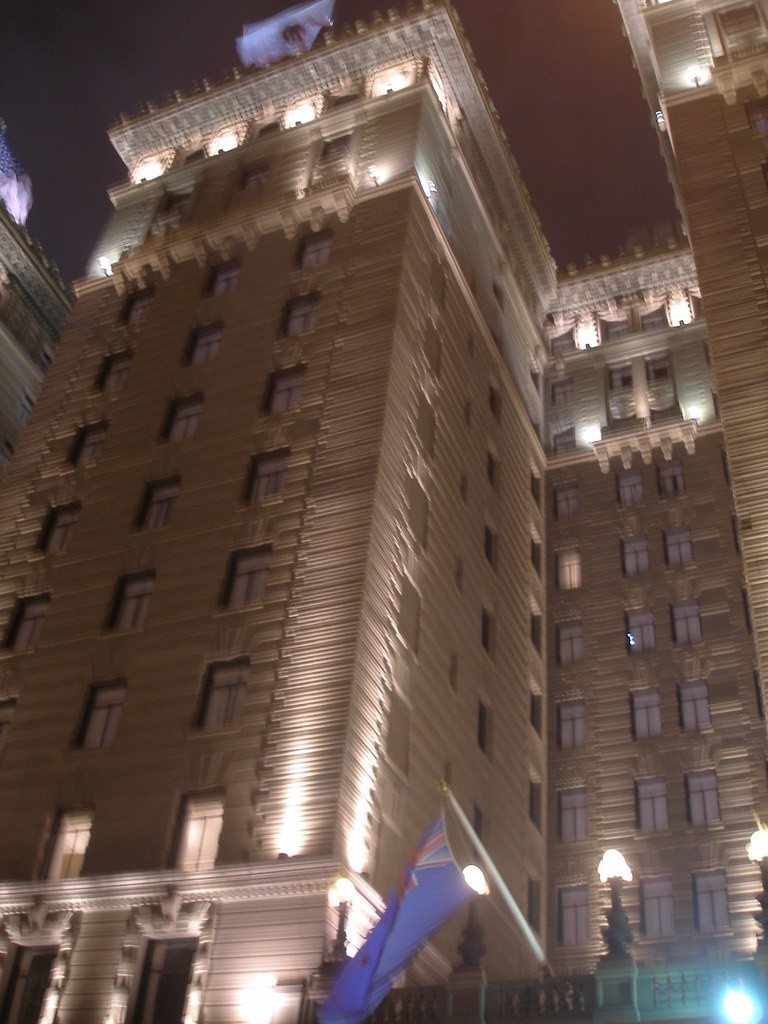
xmin=314 ymin=798 xmax=481 ymax=1024
xmin=1 ymin=128 xmax=34 ymax=225
xmin=233 ymin=0 xmax=336 ymax=68
